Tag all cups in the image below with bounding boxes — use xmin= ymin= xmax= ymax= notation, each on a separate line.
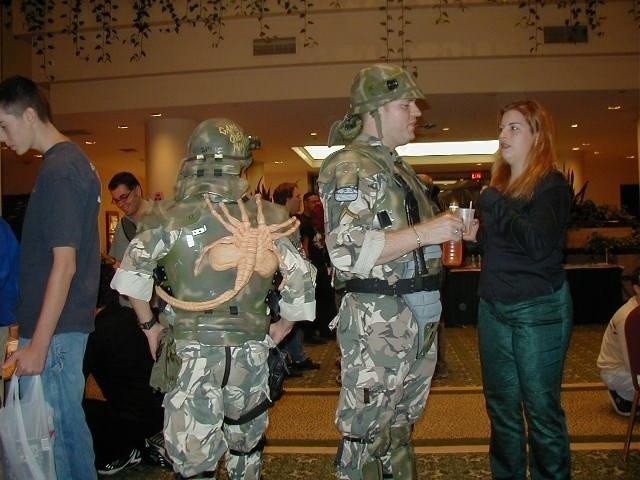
xmin=457 ymin=209 xmax=475 ymax=235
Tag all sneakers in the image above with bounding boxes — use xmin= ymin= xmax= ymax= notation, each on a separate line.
xmin=608 ymin=388 xmax=639 ymax=416
xmin=144 ymin=431 xmax=174 ymax=468
xmin=98 ymin=449 xmax=142 ymax=475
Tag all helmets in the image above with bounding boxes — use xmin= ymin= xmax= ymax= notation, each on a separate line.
xmin=175 ymin=116 xmax=261 ymax=177
xmin=349 ymin=63 xmax=427 ymax=116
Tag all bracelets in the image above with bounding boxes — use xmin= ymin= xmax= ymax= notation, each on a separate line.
xmin=413 ymin=226 xmax=420 ymax=249
xmin=140 ymin=317 xmax=156 ymax=330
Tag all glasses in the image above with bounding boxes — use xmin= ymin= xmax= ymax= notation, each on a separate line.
xmin=111 ymin=191 xmax=132 ymax=205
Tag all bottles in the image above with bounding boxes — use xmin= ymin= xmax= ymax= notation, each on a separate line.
xmin=442 ymin=199 xmax=463 ymax=269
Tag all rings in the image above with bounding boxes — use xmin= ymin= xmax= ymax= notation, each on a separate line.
xmin=456 ymin=229 xmax=458 ymax=233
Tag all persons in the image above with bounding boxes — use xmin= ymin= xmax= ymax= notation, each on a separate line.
xmin=265 ymin=183 xmax=336 ymax=377
xmin=1 ymin=74 xmax=102 ymax=480
xmin=320 ymin=62 xmax=480 ymax=479
xmin=596 ymin=267 xmax=640 ymax=417
xmin=111 ymin=118 xmax=337 ymax=477
xmin=80 ymin=263 xmax=169 ymax=474
xmin=107 ymin=171 xmax=161 ymax=324
xmin=460 ymin=99 xmax=574 ymax=480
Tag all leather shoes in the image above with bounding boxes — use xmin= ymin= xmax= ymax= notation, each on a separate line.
xmin=289 ymin=327 xmax=335 ymax=376
